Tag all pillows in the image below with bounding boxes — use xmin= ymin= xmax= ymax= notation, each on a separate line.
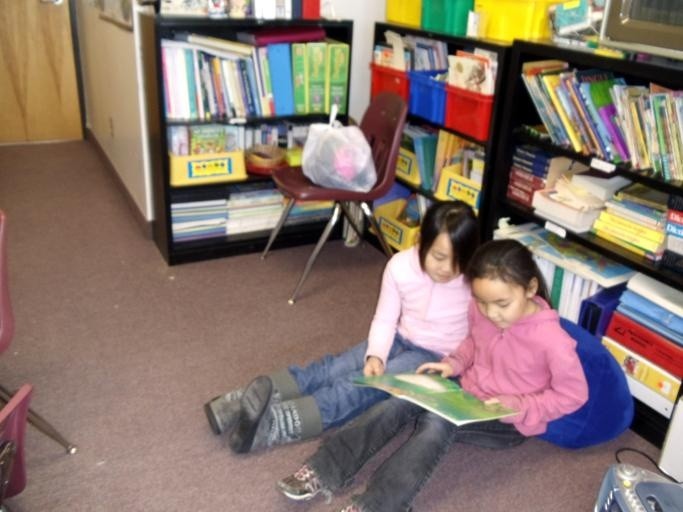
xmin=534 ymin=318 xmax=635 ymax=449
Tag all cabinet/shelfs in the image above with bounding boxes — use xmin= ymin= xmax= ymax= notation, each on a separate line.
xmin=485 ymin=36 xmax=683 ymax=451
xmin=138 ymin=11 xmax=353 ymax=266
xmin=361 ymin=19 xmax=512 ymax=256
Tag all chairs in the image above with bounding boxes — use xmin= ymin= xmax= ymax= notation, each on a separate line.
xmin=0 ymin=381 xmax=34 ymax=512
xmin=260 ymin=91 xmax=408 ymax=304
xmin=0 ymin=209 xmax=78 ymax=455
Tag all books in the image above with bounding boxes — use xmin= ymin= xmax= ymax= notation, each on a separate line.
xmin=162 ymin=0 xmax=350 ymax=243
xmin=371 ymin=31 xmax=496 ymax=224
xmin=352 ymin=373 xmax=518 ymax=425
xmin=494 ymin=1 xmax=682 ymax=417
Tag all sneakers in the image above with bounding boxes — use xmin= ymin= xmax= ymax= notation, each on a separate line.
xmin=275 ymin=465 xmax=327 ymax=501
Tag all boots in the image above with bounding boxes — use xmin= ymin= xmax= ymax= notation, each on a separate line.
xmin=227 ymin=376 xmax=322 ymax=453
xmin=203 ymin=368 xmax=300 ymax=435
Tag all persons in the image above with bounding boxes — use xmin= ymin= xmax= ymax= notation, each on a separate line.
xmin=204 ymin=199 xmax=480 ymax=451
xmin=276 ymin=238 xmax=589 ymax=512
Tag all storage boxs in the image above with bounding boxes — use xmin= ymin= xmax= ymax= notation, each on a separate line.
xmin=444 ymin=81 xmax=494 ymax=142
xmin=472 ymin=1 xmax=557 ymax=47
xmin=421 ymin=2 xmax=476 ymax=37
xmin=369 ymin=63 xmax=409 ymax=104
xmin=385 ymin=0 xmax=420 ymax=30
xmin=405 ymin=68 xmax=448 ymax=124
